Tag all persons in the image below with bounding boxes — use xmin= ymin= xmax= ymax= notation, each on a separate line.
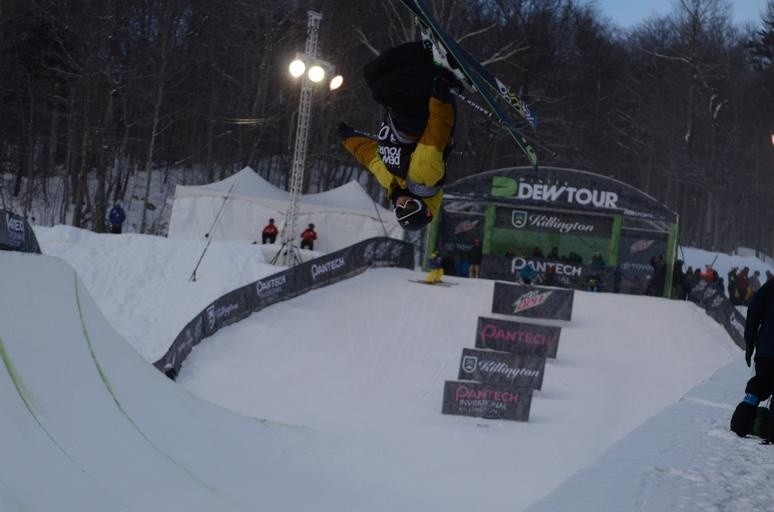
xmin=334 ymin=27 xmax=463 ymax=233
xmin=726 ymin=269 xmax=774 ymax=445
xmin=299 ymin=223 xmax=319 ymax=251
xmin=262 ymin=216 xmax=278 ymax=246
xmin=108 ymin=198 xmax=126 ymax=235
xmin=427 ymin=232 xmax=774 ymax=308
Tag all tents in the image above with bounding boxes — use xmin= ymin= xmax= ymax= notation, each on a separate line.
xmin=165 ymin=165 xmax=406 ymax=258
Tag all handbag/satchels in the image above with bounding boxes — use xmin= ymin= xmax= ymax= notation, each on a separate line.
xmin=728 ymin=400 xmax=773 ymax=443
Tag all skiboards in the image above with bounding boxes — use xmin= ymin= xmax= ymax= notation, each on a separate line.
xmin=402 ymin=0 xmax=538 ymax=172
xmin=408 ymin=277 xmax=458 ymax=287
